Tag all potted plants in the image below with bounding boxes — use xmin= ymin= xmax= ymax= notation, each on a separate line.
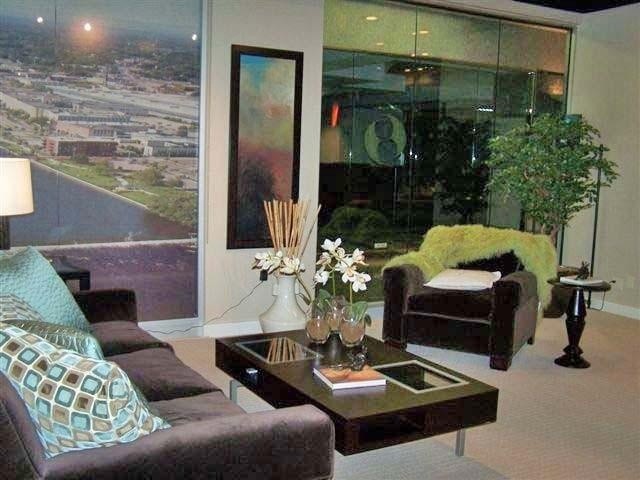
xmin=482 ymin=113 xmax=624 ymax=319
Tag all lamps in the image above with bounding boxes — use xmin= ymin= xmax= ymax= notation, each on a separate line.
xmin=0 ymin=157 xmax=35 ymax=248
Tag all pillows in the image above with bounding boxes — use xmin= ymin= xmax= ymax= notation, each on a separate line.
xmin=421 ymin=268 xmax=502 ymax=292
xmin=1 ymin=245 xmax=172 ymax=459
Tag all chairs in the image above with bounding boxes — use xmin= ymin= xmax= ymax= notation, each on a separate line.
xmin=382 ymin=225 xmax=547 ymax=373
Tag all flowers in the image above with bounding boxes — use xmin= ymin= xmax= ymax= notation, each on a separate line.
xmin=251 ymin=236 xmax=372 ymax=327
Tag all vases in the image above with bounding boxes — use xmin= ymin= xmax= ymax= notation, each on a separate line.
xmin=304 ymin=295 xmax=368 ymax=347
xmin=257 ymin=272 xmax=314 ymax=335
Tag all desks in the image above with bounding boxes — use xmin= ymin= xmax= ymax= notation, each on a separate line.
xmin=546 ymin=278 xmax=612 ymax=369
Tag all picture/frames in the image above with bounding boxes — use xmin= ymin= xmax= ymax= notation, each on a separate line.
xmin=227 ymin=44 xmax=304 ymax=249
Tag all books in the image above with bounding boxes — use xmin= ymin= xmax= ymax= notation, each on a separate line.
xmin=559 ymin=274 xmax=602 ymax=285
xmin=313 ymin=364 xmax=388 ymax=391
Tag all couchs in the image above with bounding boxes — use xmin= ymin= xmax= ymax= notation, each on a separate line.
xmin=0 ymin=285 xmax=336 ymax=479
